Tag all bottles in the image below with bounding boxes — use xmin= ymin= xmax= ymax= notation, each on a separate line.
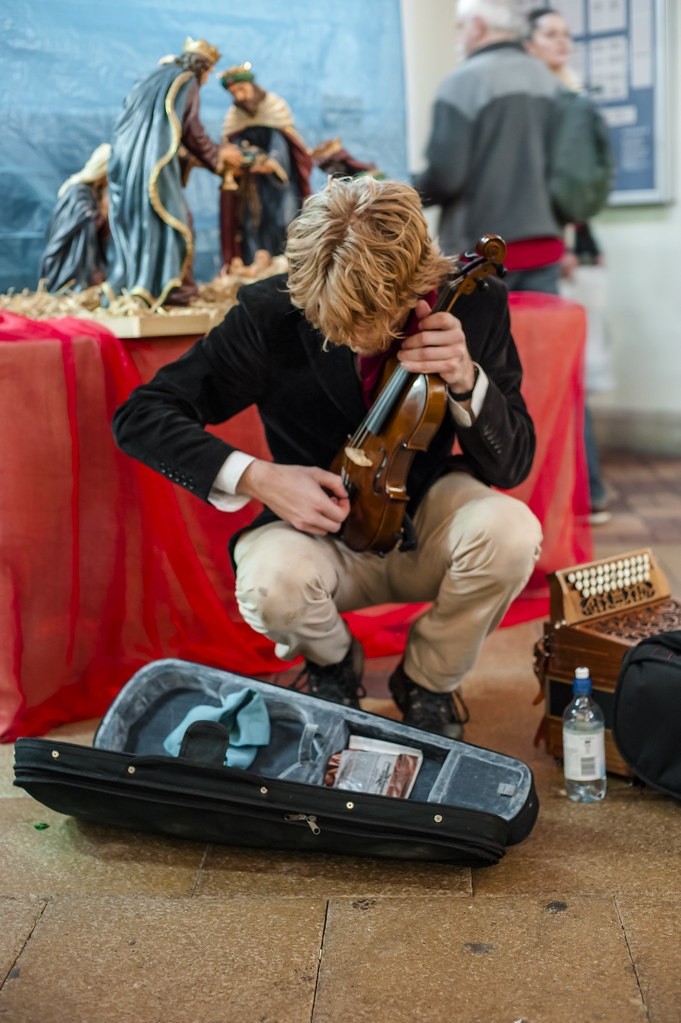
xmin=562 ymin=667 xmax=607 ymax=801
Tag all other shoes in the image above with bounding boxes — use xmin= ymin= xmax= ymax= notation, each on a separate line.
xmin=589 ymin=496 xmax=610 ymax=523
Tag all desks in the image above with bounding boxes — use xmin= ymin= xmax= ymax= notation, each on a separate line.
xmin=1 ymin=291 xmax=589 ymax=741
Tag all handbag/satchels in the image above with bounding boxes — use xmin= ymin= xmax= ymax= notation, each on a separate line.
xmin=544 ymin=83 xmax=613 ymax=231
xmin=607 ymin=631 xmax=681 ymax=799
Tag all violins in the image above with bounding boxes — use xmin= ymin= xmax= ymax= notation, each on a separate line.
xmin=328 ymin=232 xmax=509 ymax=553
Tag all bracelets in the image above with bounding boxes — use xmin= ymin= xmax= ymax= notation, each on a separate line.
xmin=446 ymin=363 xmax=479 ymax=401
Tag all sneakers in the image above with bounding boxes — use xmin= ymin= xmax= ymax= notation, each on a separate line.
xmin=387 ymin=661 xmax=469 ymax=741
xmin=288 ymin=638 xmax=366 ymax=711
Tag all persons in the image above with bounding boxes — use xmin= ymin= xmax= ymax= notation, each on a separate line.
xmin=111 ymin=177 xmax=546 ymax=744
xmin=35 ymin=37 xmax=382 ymax=306
xmin=392 ymin=0 xmax=618 ymax=524
